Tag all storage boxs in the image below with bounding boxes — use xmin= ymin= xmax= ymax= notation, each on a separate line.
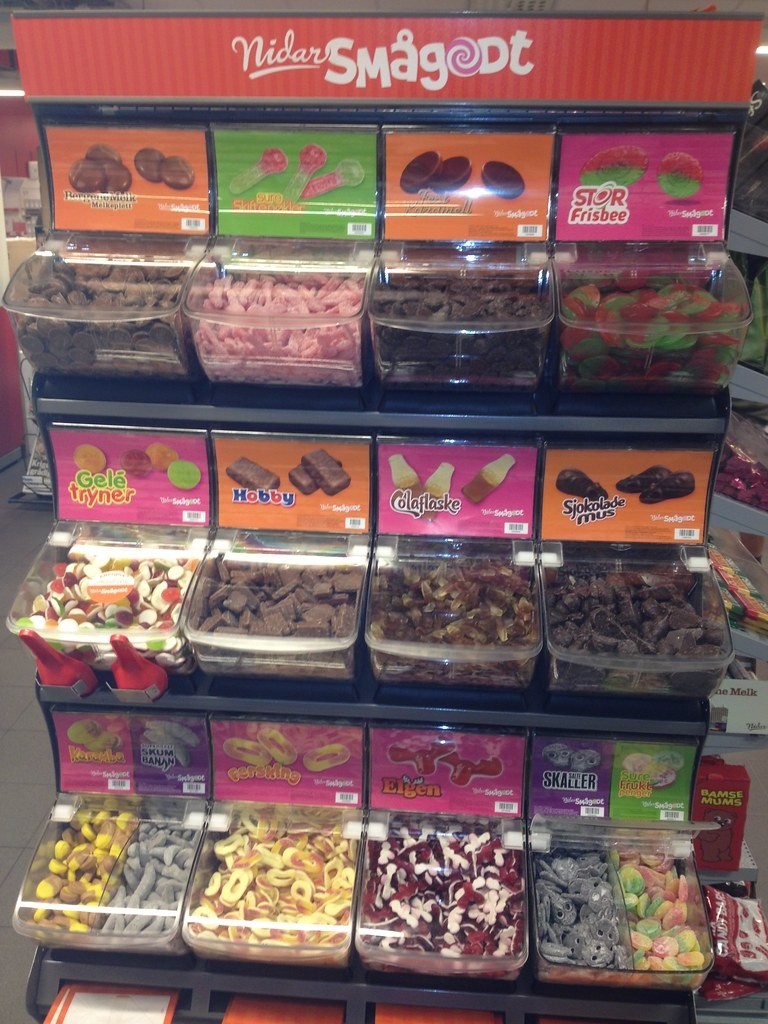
xmin=354 ymin=807 xmax=528 ymax=984
xmin=183 ymin=234 xmax=382 ymax=391
xmin=692 ymin=765 xmax=751 ymax=871
xmin=364 ymin=240 xmax=555 ymax=396
xmin=180 ymin=797 xmax=368 ymax=970
xmin=5 ymin=516 xmax=218 ymax=678
xmin=526 ymin=813 xmax=716 ymax=994
xmin=9 ymin=789 xmax=219 ymax=958
xmin=361 ymin=531 xmax=544 ymax=692
xmin=2 ymin=229 xmax=213 ymax=383
xmin=547 ymin=241 xmax=754 ymax=400
xmin=537 ymin=541 xmax=734 ymax=701
xmin=181 ymin=525 xmax=374 ymax=686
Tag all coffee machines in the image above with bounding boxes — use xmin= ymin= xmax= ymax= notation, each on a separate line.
xmin=19 ymin=180 xmax=44 ymax=237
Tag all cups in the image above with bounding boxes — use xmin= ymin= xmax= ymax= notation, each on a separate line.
xmin=28 ymin=160 xmax=39 ymax=180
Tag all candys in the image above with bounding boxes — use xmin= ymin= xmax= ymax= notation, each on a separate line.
xmin=580 ymin=146 xmax=648 ymax=187
xmin=67 ymin=719 xmax=684 ymax=787
xmin=16 ymin=556 xmax=723 ymax=689
xmin=657 ymin=152 xmax=704 ymax=198
xmin=33 ymin=799 xmax=703 ymax=982
xmin=481 ymin=162 xmax=525 ymax=199
xmin=430 ymin=157 xmax=471 ymax=193
xmin=19 ymin=261 xmax=747 ymax=391
xmin=74 ymin=445 xmax=696 ymax=522
xmin=70 ymin=144 xmax=195 ymax=194
xmin=400 ymin=152 xmax=442 ymax=192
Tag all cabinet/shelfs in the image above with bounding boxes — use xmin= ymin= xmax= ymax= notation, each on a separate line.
xmin=0 ymin=8 xmax=768 ymax=1024
xmin=694 ymin=203 xmax=768 ymax=1022
